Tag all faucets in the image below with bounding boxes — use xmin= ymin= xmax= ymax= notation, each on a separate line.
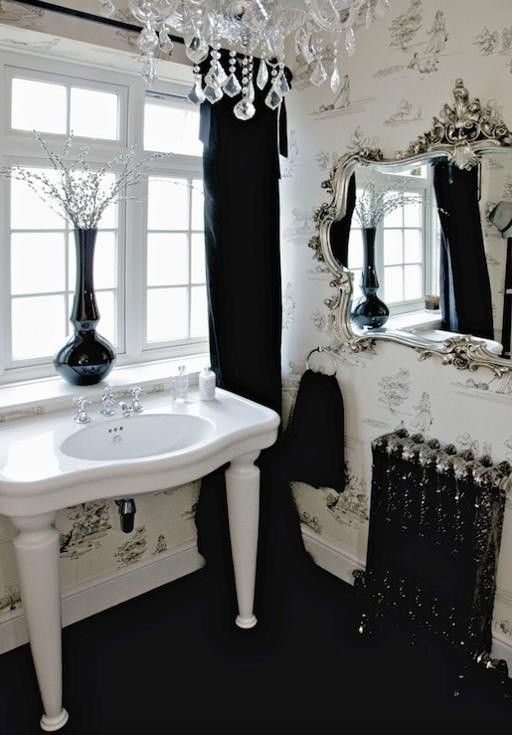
xmin=99 ymin=387 xmax=131 ymax=417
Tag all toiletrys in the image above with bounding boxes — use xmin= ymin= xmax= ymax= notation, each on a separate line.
xmin=198 ymin=363 xmax=217 ymax=402
xmin=174 ymin=364 xmax=189 ymax=402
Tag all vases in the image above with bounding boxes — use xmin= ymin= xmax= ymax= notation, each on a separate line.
xmin=352 ymin=225 xmax=389 ymax=330
xmin=53 ymin=227 xmax=117 ymax=389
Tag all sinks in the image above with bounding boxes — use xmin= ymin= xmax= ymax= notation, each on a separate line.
xmin=61 ymin=409 xmax=218 ymax=459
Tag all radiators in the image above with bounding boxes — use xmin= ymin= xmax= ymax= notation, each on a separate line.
xmin=349 ymin=428 xmax=512 ymax=686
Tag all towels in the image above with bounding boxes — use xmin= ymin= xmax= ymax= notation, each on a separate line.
xmin=277 ymin=370 xmax=349 ymax=494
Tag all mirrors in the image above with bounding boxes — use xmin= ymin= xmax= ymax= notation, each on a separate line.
xmin=305 ymin=75 xmax=512 ymax=380
